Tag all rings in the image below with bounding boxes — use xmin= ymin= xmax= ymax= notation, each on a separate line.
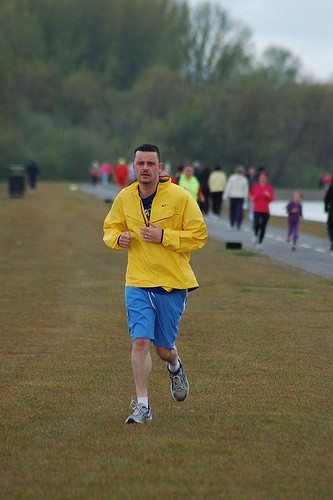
xmin=145 ymin=235 xmax=147 ymax=238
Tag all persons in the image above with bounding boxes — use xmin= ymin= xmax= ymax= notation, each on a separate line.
xmin=286 ymin=191 xmax=302 ymax=251
xmin=168 ymin=161 xmax=227 ymax=217
xmin=90 ymin=158 xmax=135 ymax=190
xmin=222 ymin=166 xmax=275 ymax=251
xmin=324 ymin=179 xmax=333 ymax=252
xmin=26 ymin=160 xmax=39 ymax=191
xmin=102 ymin=143 xmax=208 ymax=423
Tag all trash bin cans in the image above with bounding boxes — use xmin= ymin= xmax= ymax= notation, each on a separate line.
xmin=8 ymin=164 xmax=26 ymax=199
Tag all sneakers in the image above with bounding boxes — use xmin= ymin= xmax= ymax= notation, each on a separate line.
xmin=125 ymin=399 xmax=153 ymax=425
xmin=166 ymin=356 xmax=189 ymax=403
